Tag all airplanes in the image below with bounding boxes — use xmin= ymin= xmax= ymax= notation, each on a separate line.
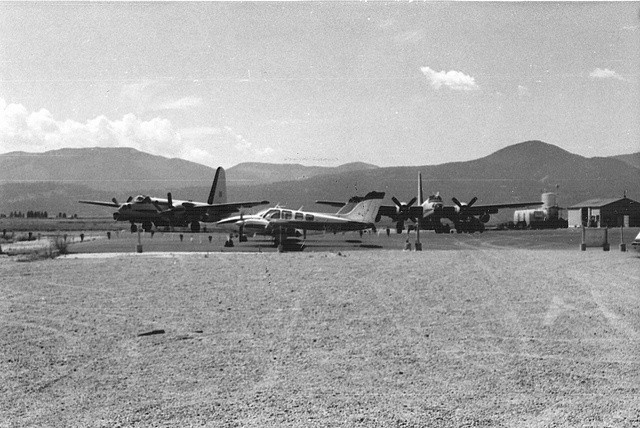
xmin=216 ymin=192 xmax=383 ymax=252
xmin=314 ymin=172 xmax=544 ymax=233
xmin=79 ymin=167 xmax=269 ymax=234
